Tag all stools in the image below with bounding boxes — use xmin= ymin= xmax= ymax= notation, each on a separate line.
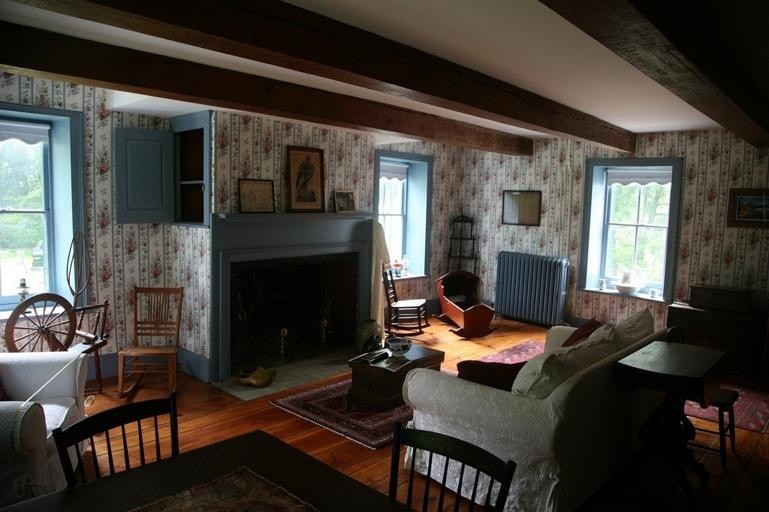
xmin=684 ymin=387 xmax=744 ymax=474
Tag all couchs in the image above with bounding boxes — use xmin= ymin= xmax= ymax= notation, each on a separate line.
xmin=398 ymin=323 xmax=675 ymax=511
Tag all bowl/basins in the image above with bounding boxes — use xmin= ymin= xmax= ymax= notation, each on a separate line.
xmin=385 ymin=337 xmax=413 ymax=358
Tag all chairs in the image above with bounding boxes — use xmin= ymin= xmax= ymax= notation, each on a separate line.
xmin=381 ymin=269 xmax=432 ymax=337
xmin=0 ymin=351 xmax=90 ymax=507
xmin=115 ymin=285 xmax=185 ymax=417
xmin=52 ymin=389 xmax=181 ymax=487
xmin=385 ymin=419 xmax=518 ymax=512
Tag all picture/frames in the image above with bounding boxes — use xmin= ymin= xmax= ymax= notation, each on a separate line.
xmin=501 ymin=189 xmax=542 ymax=228
xmin=725 ymin=186 xmax=769 ymax=231
xmin=334 ymin=189 xmax=358 ymax=215
xmin=284 ymin=144 xmax=329 ymax=214
xmin=236 ymin=177 xmax=277 ymax=216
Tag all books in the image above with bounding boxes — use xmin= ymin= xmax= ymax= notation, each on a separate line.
xmin=348 ymin=351 xmax=412 ymax=372
xmin=391 ymin=261 xmax=409 ymax=278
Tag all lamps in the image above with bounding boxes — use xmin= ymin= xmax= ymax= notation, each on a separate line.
xmin=15 ymin=278 xmax=33 ymax=314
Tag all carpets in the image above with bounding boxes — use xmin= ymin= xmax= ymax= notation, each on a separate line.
xmin=475 ymin=338 xmax=769 ymax=435
xmin=267 ymin=365 xmax=459 ymax=451
xmin=682 ymin=377 xmax=769 ymax=436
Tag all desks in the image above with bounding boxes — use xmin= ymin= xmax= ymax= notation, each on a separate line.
xmin=617 ymin=340 xmax=728 ymax=471
xmin=666 ymin=281 xmax=768 ymax=386
xmin=4 ymin=429 xmax=418 ymax=510
xmin=346 ymin=343 xmax=445 ymax=413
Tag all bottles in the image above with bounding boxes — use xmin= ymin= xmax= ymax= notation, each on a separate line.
xmin=392 ymin=254 xmax=408 ymax=278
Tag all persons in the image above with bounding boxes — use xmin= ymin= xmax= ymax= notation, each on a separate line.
xmin=337 ymin=194 xmax=346 ymax=209
xmin=346 ymin=193 xmax=353 ymax=211
xmin=738 ymin=203 xmax=754 ymax=219
xmin=295 ymin=155 xmax=316 ymax=195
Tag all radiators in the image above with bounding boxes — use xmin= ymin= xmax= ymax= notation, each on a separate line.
xmin=492 ymin=250 xmax=572 ymax=330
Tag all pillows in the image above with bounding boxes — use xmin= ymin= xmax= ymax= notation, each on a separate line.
xmin=455 ymin=358 xmax=528 ymax=391
xmin=614 ymin=305 xmax=657 ymax=348
xmin=510 ymin=322 xmax=617 ymax=401
xmin=561 ymin=317 xmax=603 ymax=348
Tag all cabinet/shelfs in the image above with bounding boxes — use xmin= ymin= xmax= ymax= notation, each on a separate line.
xmin=446 ymin=202 xmax=478 ymax=275
xmin=111 ymin=109 xmax=216 ymax=229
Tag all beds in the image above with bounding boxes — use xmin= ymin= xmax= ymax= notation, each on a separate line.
xmin=433 ymin=269 xmax=498 ymax=340
